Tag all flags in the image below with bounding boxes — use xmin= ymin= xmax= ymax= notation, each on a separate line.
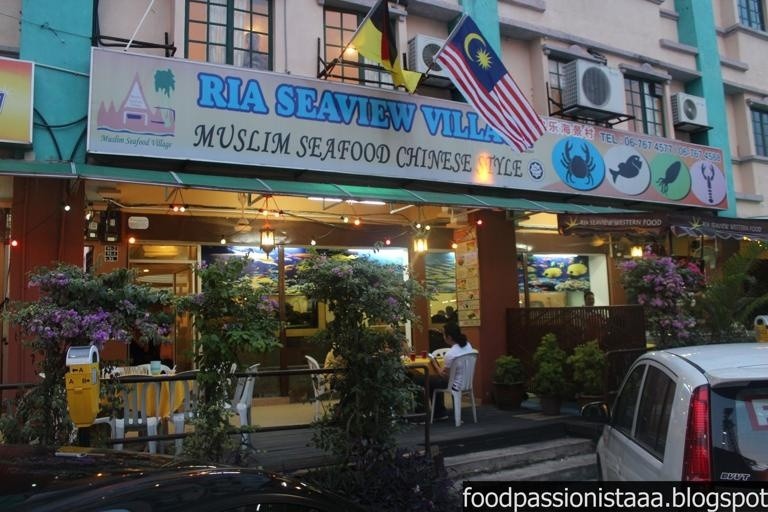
xmin=436 ymin=12 xmax=546 ymax=155
xmin=350 ymin=1 xmax=425 ymax=95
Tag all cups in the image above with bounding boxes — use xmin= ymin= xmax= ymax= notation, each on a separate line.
xmin=409 ymin=347 xmax=416 ymax=362
xmin=421 ymin=348 xmax=426 ymax=358
xmin=151 ymin=360 xmax=161 ymax=375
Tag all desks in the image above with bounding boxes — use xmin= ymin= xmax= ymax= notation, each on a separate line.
xmin=102 ymin=375 xmax=199 ymax=419
xmin=405 ymin=355 xmax=445 ymax=375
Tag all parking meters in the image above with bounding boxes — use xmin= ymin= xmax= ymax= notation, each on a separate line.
xmin=754 ymin=316 xmax=768 ymax=343
xmin=64 ymin=345 xmax=100 ymax=446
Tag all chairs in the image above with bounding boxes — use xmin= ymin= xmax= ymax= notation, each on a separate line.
xmin=138 ymin=364 xmax=170 ymax=374
xmin=430 ymin=352 xmax=480 ymax=427
xmin=219 ymin=363 xmax=261 ymax=452
xmin=70 ymin=411 xmax=116 ymax=448
xmin=165 ymin=369 xmax=210 ymax=457
xmin=432 ymin=348 xmax=451 ymax=357
xmin=102 ymin=365 xmax=124 ymax=375
xmin=114 ymin=374 xmax=165 ymax=456
xmin=304 ymin=354 xmax=338 ymax=421
xmin=217 ymin=361 xmax=238 ymax=402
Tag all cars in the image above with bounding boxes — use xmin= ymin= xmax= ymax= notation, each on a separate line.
xmin=579 ymin=341 xmax=768 ymax=481
xmin=0 ymin=442 xmax=378 ymax=512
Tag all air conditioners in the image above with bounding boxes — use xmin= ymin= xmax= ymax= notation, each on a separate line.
xmin=407 ymin=32 xmax=452 ymax=86
xmin=561 ymin=59 xmax=626 ymax=119
xmin=670 ymin=92 xmax=708 ymax=131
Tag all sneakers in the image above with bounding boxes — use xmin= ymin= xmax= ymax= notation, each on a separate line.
xmin=411 ymin=417 xmax=426 ymax=424
xmin=434 ymin=411 xmax=449 ymax=420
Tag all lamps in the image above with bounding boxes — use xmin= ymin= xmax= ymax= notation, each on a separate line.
xmin=260 ymin=194 xmax=276 ymax=258
xmin=412 ymin=201 xmax=430 ymax=260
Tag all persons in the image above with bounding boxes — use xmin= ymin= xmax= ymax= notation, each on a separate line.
xmin=320 ymin=341 xmax=353 ymax=391
xmin=432 ymin=309 xmax=447 ymax=322
xmin=444 ymin=305 xmax=460 ymax=322
xmin=416 ymin=323 xmax=475 ymax=424
xmin=575 ymin=291 xmax=605 ymax=323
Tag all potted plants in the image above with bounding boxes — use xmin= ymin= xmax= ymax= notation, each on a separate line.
xmin=532 ymin=331 xmax=567 ymax=415
xmin=490 ymin=354 xmax=526 ymax=410
xmin=568 ymin=339 xmax=609 ymax=415
